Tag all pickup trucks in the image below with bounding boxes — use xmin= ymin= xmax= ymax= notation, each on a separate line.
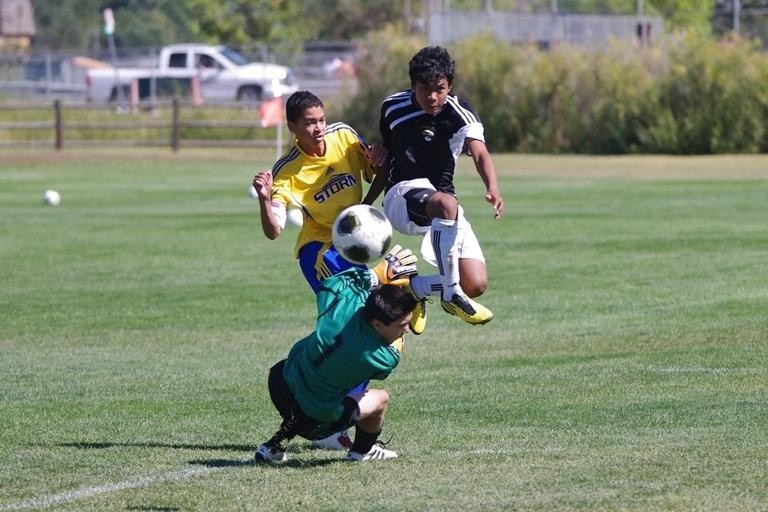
xmin=84 ymin=39 xmax=298 ymax=106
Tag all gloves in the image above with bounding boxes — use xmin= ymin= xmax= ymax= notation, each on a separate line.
xmin=367 ymin=244 xmax=418 ymax=286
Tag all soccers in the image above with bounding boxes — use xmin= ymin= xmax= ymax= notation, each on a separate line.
xmin=332 ymin=204 xmax=393 ymax=265
xmin=45 ymin=191 xmax=59 ymax=205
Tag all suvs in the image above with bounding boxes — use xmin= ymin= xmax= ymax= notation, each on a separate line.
xmin=285 ymin=38 xmax=369 ymax=88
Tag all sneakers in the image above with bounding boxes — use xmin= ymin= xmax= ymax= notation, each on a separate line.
xmin=399 ymin=273 xmax=433 ymax=336
xmin=346 ymin=440 xmax=398 ymax=463
xmin=311 ymin=430 xmax=353 ymax=450
xmin=440 ymin=289 xmax=494 ymax=326
xmin=254 ymin=442 xmax=288 ymax=462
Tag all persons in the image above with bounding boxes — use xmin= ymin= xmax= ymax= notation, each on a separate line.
xmin=253 ymin=91 xmax=388 ymax=449
xmin=363 ymin=46 xmax=503 ymax=335
xmin=255 ymin=245 xmax=416 ymax=466
